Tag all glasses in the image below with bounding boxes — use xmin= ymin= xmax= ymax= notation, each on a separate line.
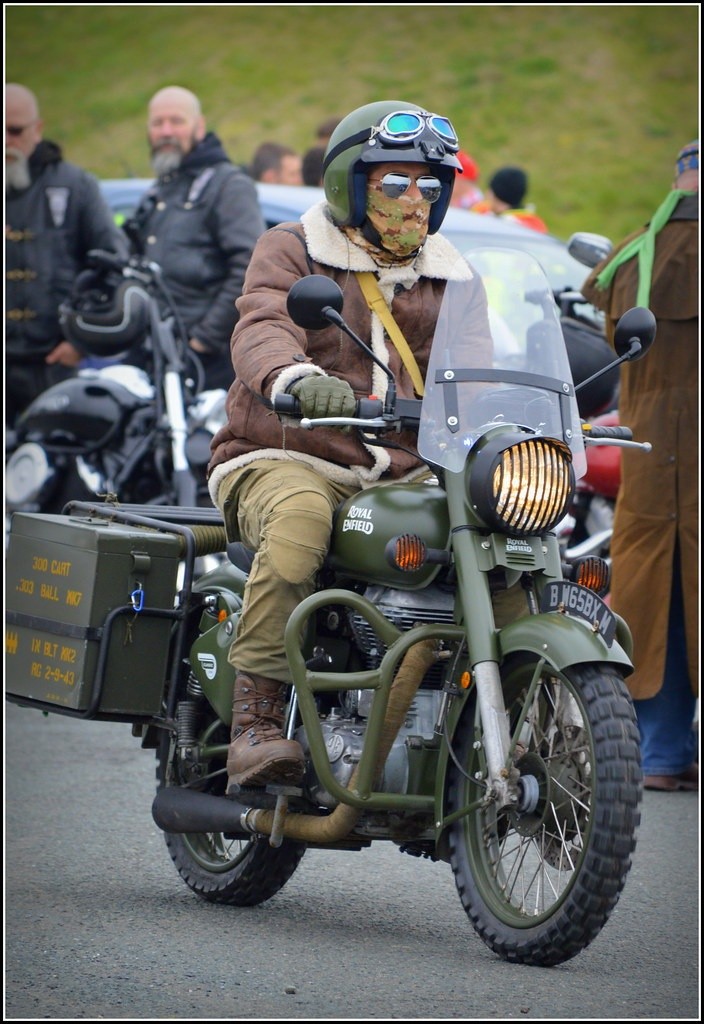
xmin=376 ymin=110 xmax=460 ymax=153
xmin=8 ymin=120 xmax=37 ymax=137
xmin=368 ymin=171 xmax=443 ymax=203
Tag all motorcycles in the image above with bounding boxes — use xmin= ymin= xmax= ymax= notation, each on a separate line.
xmin=6 ymin=272 xmax=657 ymax=971
xmin=4 ymin=244 xmax=240 ymax=714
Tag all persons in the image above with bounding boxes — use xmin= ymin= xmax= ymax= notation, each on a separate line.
xmin=580 ymin=141 xmax=699 ymax=790
xmin=119 ymin=87 xmax=265 ymax=399
xmin=5 ymin=82 xmax=128 ymax=432
xmin=249 ymin=118 xmax=343 ymax=187
xmin=207 ymin=100 xmax=501 ymax=792
xmin=448 ymin=152 xmax=547 ymax=233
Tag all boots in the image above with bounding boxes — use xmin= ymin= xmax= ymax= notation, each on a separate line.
xmin=225 ymin=671 xmax=305 ymax=800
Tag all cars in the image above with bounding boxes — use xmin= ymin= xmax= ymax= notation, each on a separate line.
xmin=86 ymin=175 xmax=617 ymax=365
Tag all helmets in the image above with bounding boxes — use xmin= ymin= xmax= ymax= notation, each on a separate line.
xmin=58 ymin=270 xmax=151 ymax=362
xmin=321 ymin=100 xmax=464 ymax=235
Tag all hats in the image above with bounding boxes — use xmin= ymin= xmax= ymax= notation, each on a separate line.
xmin=673 ymin=141 xmax=699 ymax=176
xmin=491 ymin=168 xmax=527 ymax=204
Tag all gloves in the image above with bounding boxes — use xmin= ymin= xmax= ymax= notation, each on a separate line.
xmin=286 ymin=372 xmax=356 ymax=437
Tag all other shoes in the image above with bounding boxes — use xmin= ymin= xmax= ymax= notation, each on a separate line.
xmin=643 ymin=761 xmax=697 ymax=791
